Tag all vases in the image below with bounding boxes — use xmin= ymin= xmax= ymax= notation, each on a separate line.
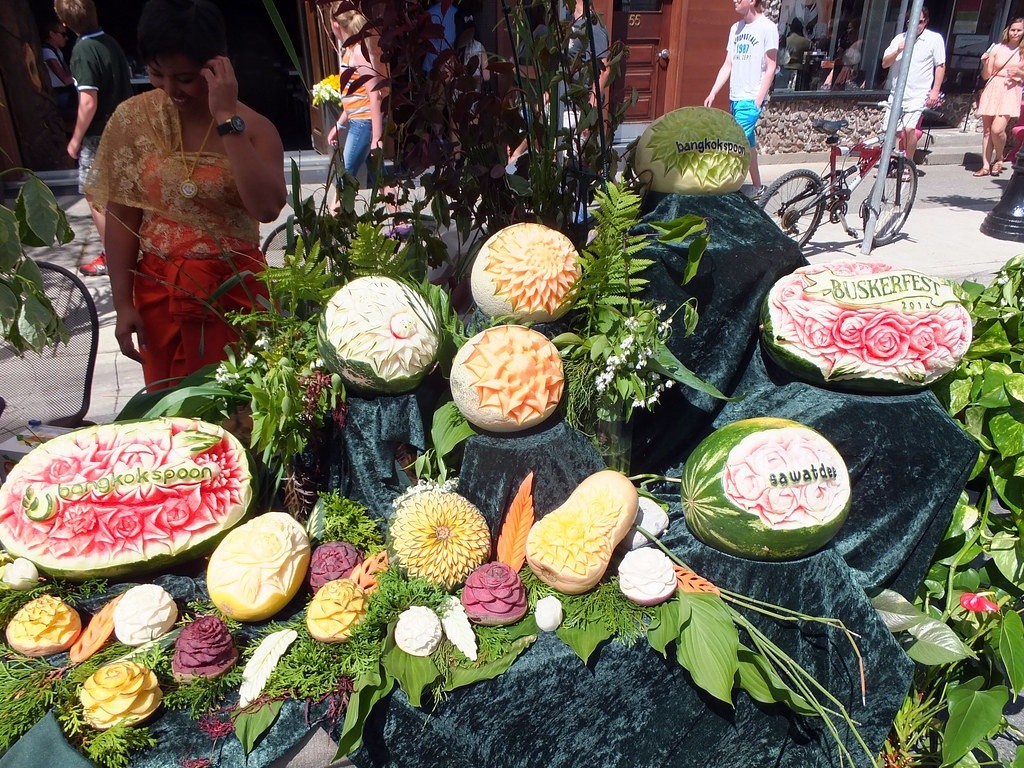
xmin=322 ymin=102 xmax=349 ymax=159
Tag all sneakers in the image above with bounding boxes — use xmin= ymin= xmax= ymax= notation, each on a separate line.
xmin=79 ymin=252 xmax=110 ymax=275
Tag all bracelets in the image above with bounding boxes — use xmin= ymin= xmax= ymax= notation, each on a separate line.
xmin=337 ymin=122 xmax=346 ymax=128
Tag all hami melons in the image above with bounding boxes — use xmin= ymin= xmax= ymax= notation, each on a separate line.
xmin=206 ymin=509 xmax=311 ymax=622
xmin=471 ymin=222 xmax=582 ymax=324
xmin=634 ymin=105 xmax=751 ymax=194
xmin=387 ymin=491 xmax=491 ymax=592
xmin=449 ymin=324 xmax=565 ymax=431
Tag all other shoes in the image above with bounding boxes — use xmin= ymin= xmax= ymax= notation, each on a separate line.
xmin=901 ymin=168 xmax=910 ymax=182
xmin=383 ymin=225 xmax=414 ymax=243
xmin=872 ymin=166 xmax=898 ymax=178
xmin=973 ymin=168 xmax=990 ymax=176
xmin=745 ymin=185 xmax=767 ymax=200
xmin=991 ymin=157 xmax=1004 ymax=175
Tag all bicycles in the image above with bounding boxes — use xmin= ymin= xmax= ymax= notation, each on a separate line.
xmin=756 ymin=100 xmax=944 ymax=250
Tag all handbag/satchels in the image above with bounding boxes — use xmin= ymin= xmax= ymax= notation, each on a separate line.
xmin=976 ymin=89 xmax=983 ymax=108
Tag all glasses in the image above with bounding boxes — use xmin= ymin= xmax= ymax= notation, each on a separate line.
xmin=56 ymin=31 xmax=67 ymax=37
xmin=906 ymin=19 xmax=927 ymax=25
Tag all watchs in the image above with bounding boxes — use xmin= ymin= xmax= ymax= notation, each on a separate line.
xmin=215 ymin=116 xmax=244 ymax=135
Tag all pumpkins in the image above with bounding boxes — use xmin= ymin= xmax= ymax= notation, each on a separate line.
xmin=527 ymin=470 xmax=638 ymax=596
xmin=79 ymin=660 xmax=162 ymax=730
xmin=5 ymin=595 xmax=82 ymax=659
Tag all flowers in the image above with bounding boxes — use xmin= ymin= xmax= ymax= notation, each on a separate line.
xmin=635 ymin=106 xmax=750 ymax=195
xmin=205 ymin=237 xmax=347 ymax=471
xmin=0 ymin=222 xmax=677 ymax=728
xmin=680 ymin=418 xmax=852 ymax=561
xmin=759 ymin=260 xmax=973 ymax=395
xmin=311 ymin=74 xmax=343 ymax=108
xmin=551 ymin=173 xmax=749 ymax=424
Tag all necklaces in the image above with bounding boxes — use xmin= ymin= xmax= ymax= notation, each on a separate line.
xmin=179 ymin=120 xmax=213 ymax=199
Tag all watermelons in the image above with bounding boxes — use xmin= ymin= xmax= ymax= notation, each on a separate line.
xmin=318 ymin=275 xmax=442 ymax=395
xmin=0 ymin=416 xmax=258 ymax=581
xmin=681 ymin=416 xmax=851 ymax=561
xmin=759 ymin=263 xmax=973 ymax=392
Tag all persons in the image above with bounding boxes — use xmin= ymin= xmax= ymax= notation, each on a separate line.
xmin=505 ymin=0 xmax=607 ymax=240
xmin=54 ymin=0 xmax=132 ymax=275
xmin=704 ymin=0 xmax=780 ymax=200
xmin=776 ymin=0 xmax=861 ymax=68
xmin=421 ymin=0 xmax=498 ymax=172
xmin=974 ymin=17 xmax=1024 ymax=176
xmin=80 ymin=1 xmax=288 ymax=394
xmin=42 ymin=16 xmax=76 ymax=105
xmin=327 ymin=0 xmax=400 ymax=217
xmin=872 ymin=8 xmax=945 ymax=182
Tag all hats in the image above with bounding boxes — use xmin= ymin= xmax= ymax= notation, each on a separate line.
xmin=789 ymin=17 xmax=804 ymax=28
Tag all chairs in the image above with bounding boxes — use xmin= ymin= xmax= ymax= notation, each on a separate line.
xmin=261 ymin=219 xmax=349 ymax=288
xmin=0 ymin=261 xmax=98 ymax=428
